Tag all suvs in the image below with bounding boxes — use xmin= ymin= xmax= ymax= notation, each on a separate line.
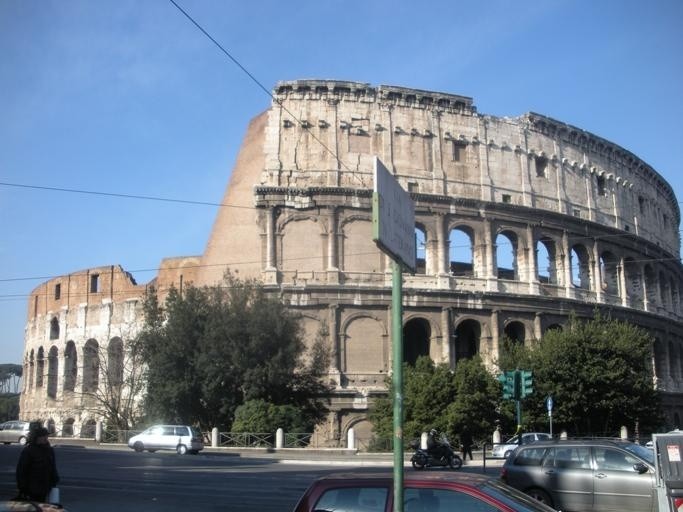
xmin=0 ymin=420 xmax=31 ymax=446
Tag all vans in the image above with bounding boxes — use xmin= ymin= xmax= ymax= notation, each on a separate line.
xmin=127 ymin=424 xmax=204 ymax=455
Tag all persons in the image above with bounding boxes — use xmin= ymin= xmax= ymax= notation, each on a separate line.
xmin=460 ymin=425 xmax=474 ymax=460
xmin=426 ymin=429 xmax=448 ymax=461
xmin=15 ymin=427 xmax=60 ymax=503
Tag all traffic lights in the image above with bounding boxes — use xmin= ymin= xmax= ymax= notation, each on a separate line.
xmin=499 ymin=371 xmax=516 ymax=400
xmin=518 ymin=369 xmax=533 ymax=399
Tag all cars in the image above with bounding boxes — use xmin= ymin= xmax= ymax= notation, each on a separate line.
xmin=491 ymin=429 xmax=682 ymax=512
xmin=0 ymin=500 xmax=72 ymax=512
xmin=290 ymin=470 xmax=562 ymax=512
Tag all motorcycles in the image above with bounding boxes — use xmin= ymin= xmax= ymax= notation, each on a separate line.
xmin=408 ymin=438 xmax=465 ymax=470
